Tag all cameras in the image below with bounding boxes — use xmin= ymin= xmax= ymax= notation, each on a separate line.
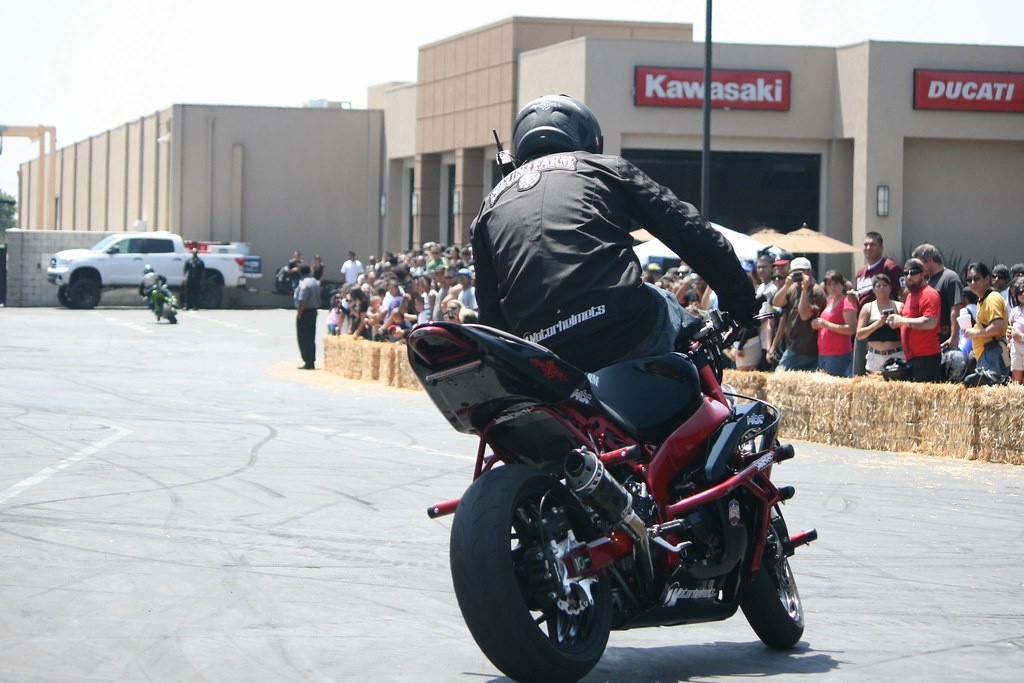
xmin=791 ymin=271 xmax=803 ymax=283
xmin=446 ymin=307 xmax=455 ymax=319
xmin=690 ymin=273 xmax=698 ymax=284
xmin=883 ymin=308 xmax=894 ymax=318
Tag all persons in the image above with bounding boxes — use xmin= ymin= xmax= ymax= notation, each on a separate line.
xmin=294 ymin=265 xmax=322 ymax=371
xmin=182 ymin=249 xmax=205 ymax=311
xmin=639 ymin=232 xmax=1024 ymax=387
xmin=139 ymin=264 xmax=178 ymax=320
xmin=469 ymin=93 xmax=761 ymax=579
xmin=274 ymin=242 xmax=477 ymax=345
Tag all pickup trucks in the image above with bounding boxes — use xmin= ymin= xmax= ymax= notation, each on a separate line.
xmin=46 ymin=228 xmax=245 ymax=309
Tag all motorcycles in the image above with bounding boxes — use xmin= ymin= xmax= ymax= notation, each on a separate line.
xmin=404 ymin=291 xmax=818 ymax=683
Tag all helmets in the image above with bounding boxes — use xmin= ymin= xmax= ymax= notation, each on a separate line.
xmin=144 ymin=266 xmax=153 ymax=274
xmin=512 ymin=94 xmax=604 ymax=167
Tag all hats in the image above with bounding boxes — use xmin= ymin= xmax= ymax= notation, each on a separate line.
xmin=771 ymin=252 xmax=794 ymax=265
xmin=457 ymin=269 xmax=472 ymax=278
xmin=788 ymin=257 xmax=812 ymax=272
xmin=433 ymin=265 xmax=446 ymax=273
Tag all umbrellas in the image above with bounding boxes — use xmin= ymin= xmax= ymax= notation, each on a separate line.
xmin=628 ymin=223 xmax=862 ymax=267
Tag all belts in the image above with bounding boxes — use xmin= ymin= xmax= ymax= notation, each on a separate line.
xmin=867 ymin=346 xmax=903 ymax=356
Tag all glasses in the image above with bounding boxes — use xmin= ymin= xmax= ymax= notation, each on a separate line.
xmin=1018 ymin=288 xmax=1024 ymax=295
xmin=991 ymin=274 xmax=1006 ymax=279
xmin=903 ymin=270 xmax=923 ymax=276
xmin=771 ymin=274 xmax=789 ymax=280
xmin=966 ymin=277 xmax=980 ymax=282
xmin=445 ymin=275 xmax=456 ymax=279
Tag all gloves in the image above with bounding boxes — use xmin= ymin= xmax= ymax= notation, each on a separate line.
xmin=727 ymin=297 xmax=762 ymax=328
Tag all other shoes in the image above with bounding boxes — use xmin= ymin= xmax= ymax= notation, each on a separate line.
xmin=298 ymin=363 xmax=314 ymax=369
